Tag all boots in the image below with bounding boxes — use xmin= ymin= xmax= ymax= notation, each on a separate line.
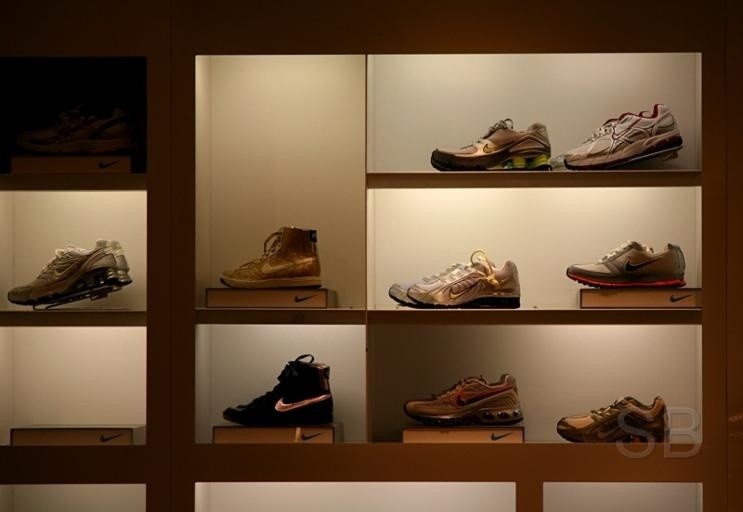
xmin=220 ymin=226 xmax=323 ymax=288
xmin=222 ymin=353 xmax=334 ymax=426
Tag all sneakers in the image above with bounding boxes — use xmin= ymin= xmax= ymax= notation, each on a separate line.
xmin=389 ymin=249 xmax=521 ymax=309
xmin=432 ymin=118 xmax=552 ymax=171
xmin=17 ymin=107 xmax=130 ymax=153
xmin=563 ymin=103 xmax=682 ymax=170
xmin=403 ymin=373 xmax=524 ymax=427
xmin=567 ymin=240 xmax=686 ymax=288
xmin=556 ymin=395 xmax=671 ymax=443
xmin=8 ymin=239 xmax=132 ymax=304
